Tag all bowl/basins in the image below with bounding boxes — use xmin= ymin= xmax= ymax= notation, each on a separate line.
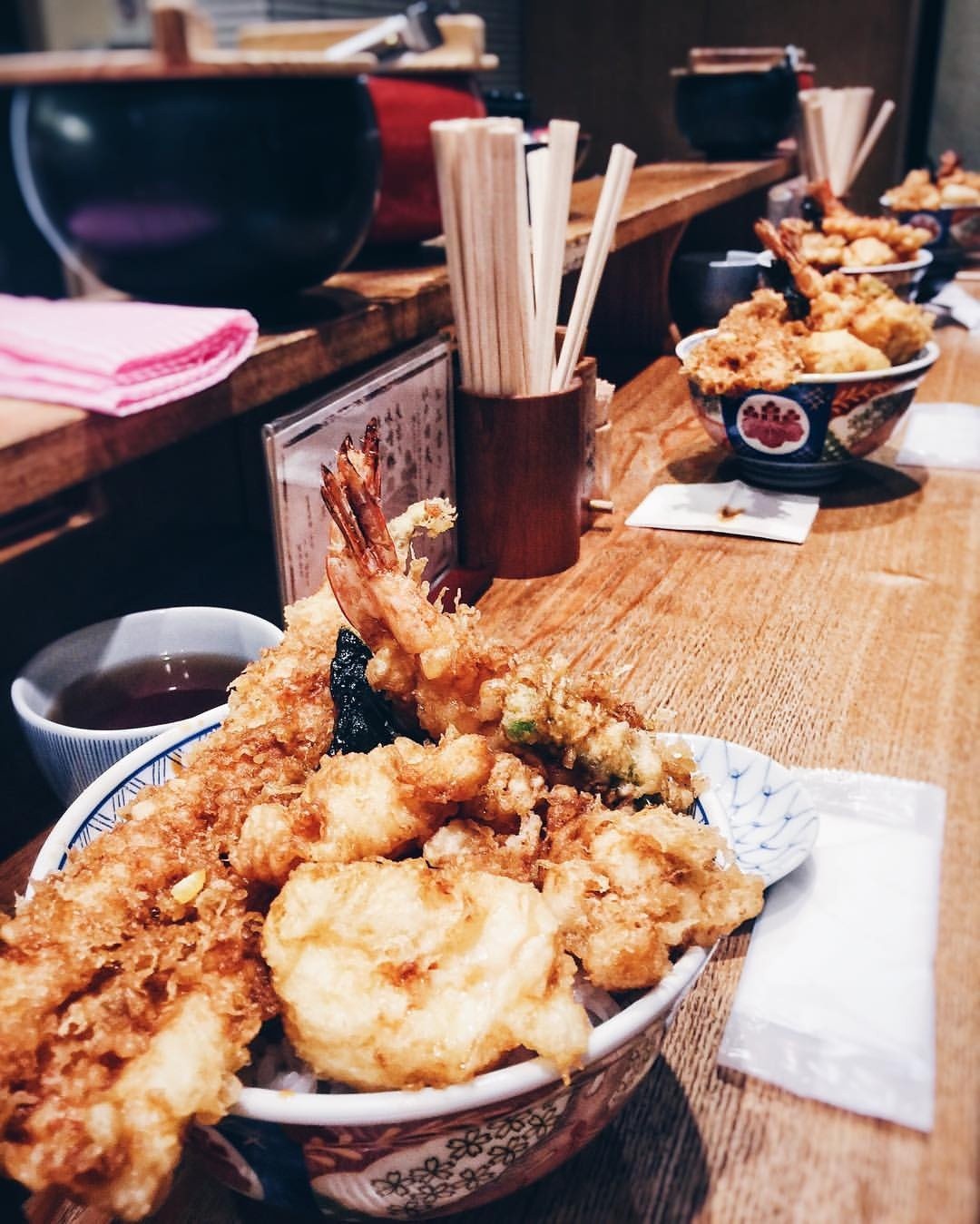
xmin=525 ymin=128 xmax=591 ymax=178
xmin=30 ymin=703 xmax=730 ymax=1223
xmin=759 ymin=250 xmax=935 ymax=303
xmin=674 ymin=71 xmax=798 ymax=160
xmin=879 ymin=195 xmax=979 ymax=261
xmin=673 ymin=330 xmax=939 ymax=489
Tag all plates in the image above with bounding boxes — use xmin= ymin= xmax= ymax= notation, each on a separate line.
xmin=650 ymin=732 xmax=820 ymax=885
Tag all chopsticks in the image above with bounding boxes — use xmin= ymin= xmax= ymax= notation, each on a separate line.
xmin=432 ymin=117 xmax=637 ymax=395
xmin=797 ymin=85 xmax=895 ymax=199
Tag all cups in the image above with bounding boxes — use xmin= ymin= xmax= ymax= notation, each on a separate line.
xmin=682 ymin=248 xmax=762 ymax=324
xmin=8 ymin=605 xmax=285 ymax=809
xmin=453 ymin=380 xmax=582 ymax=579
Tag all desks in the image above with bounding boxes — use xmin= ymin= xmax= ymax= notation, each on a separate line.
xmin=22 ymin=268 xmax=980 ymax=1224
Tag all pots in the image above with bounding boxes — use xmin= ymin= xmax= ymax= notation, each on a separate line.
xmin=238 ymin=15 xmax=500 ymax=241
xmin=0 ymin=10 xmax=390 ymax=301
